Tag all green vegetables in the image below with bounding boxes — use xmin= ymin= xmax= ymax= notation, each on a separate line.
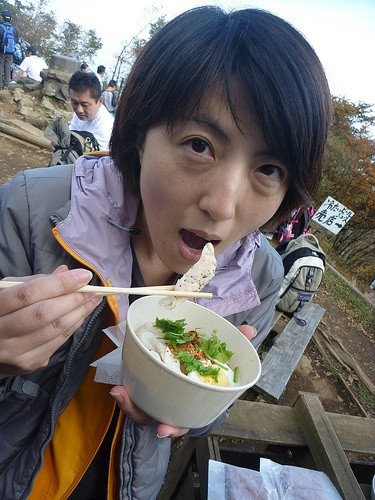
xmin=152 ymin=317 xmax=240 ymax=383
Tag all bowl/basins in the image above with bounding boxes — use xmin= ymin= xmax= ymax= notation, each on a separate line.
xmin=121 ymin=295 xmax=262 ymax=430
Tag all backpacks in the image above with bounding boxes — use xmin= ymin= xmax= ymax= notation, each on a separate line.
xmin=273 ymin=233 xmax=326 ymax=319
xmin=0 ymin=23 xmax=16 ymax=54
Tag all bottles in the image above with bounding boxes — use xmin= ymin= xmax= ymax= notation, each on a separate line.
xmin=284 ymin=224 xmax=291 ymax=234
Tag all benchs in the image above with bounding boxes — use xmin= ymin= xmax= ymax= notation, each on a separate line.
xmin=239 ymin=300 xmax=326 ymax=402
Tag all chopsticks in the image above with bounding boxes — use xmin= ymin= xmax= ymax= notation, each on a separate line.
xmin=0 ymin=280 xmax=214 ymax=299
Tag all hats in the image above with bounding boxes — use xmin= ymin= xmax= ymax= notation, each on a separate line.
xmin=26 ymin=46 xmax=36 ymax=55
xmin=2 ymin=10 xmax=11 ymax=22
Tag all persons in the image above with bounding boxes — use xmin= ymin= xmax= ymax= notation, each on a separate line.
xmin=81 ymin=64 xmax=89 ymax=72
xmin=96 ymin=65 xmax=118 ymax=114
xmin=70 ymin=71 xmax=115 ymax=153
xmin=0 ymin=4 xmax=333 ymax=500
xmin=14 ymin=46 xmax=48 ymax=84
xmin=0 ymin=11 xmax=18 ymax=90
